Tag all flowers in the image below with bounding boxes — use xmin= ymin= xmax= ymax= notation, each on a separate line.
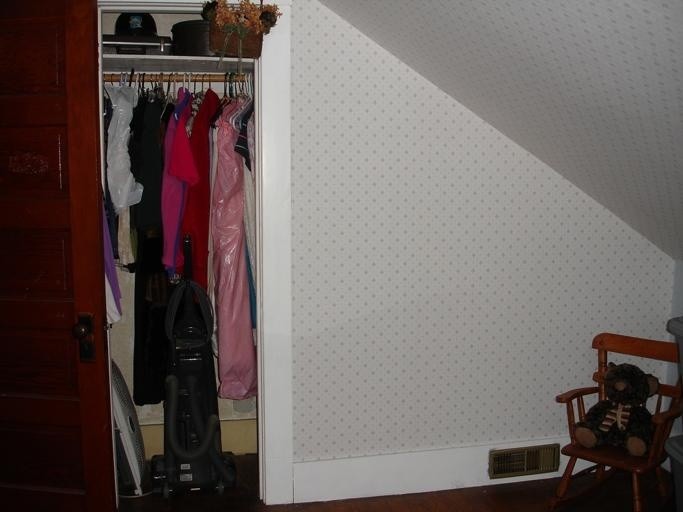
xmin=214 ymin=0 xmax=283 ymax=40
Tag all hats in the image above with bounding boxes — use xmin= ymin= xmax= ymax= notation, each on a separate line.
xmin=102 ymin=13 xmax=170 ymax=43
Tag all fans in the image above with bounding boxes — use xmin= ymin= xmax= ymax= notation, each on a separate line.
xmin=110 ymin=359 xmax=146 ymax=496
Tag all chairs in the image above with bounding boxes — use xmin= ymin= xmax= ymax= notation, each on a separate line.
xmin=553 ymin=333 xmax=683 ymax=512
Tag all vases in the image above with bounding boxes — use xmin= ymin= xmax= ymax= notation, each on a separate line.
xmin=210 ymin=19 xmax=263 ymax=59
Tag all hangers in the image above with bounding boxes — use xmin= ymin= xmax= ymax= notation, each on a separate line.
xmin=102 ymin=73 xmax=254 ymax=133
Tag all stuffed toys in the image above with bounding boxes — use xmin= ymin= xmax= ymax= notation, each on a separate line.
xmin=573 ymin=361 xmax=660 ymax=458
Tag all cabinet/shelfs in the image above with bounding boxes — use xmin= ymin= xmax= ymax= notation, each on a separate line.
xmin=98 ymin=0 xmax=263 ymax=511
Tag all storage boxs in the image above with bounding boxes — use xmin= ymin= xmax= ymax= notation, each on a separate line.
xmin=667 ymin=315 xmax=683 ymax=381
xmin=664 ymin=434 xmax=683 ymax=512
